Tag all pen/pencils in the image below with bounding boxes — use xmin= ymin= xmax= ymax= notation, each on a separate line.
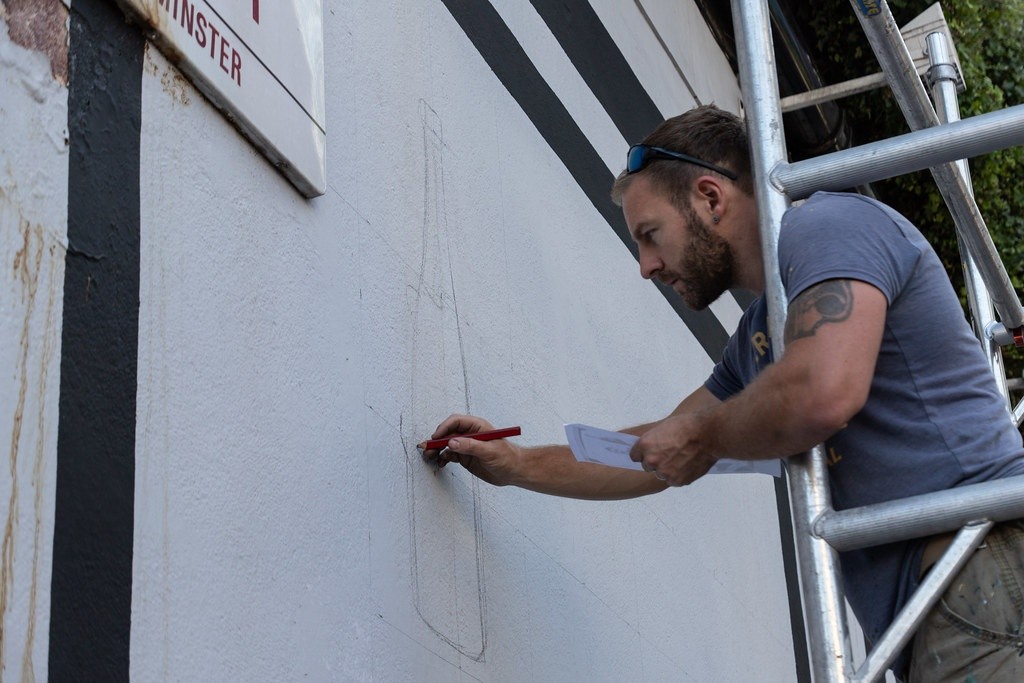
xmin=417 ymin=425 xmax=521 ymax=449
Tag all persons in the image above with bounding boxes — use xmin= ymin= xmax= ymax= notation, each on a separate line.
xmin=422 ymin=102 xmax=1024 ymax=683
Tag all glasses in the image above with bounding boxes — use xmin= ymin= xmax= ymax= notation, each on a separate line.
xmin=627 ymin=143 xmax=737 ymax=180
